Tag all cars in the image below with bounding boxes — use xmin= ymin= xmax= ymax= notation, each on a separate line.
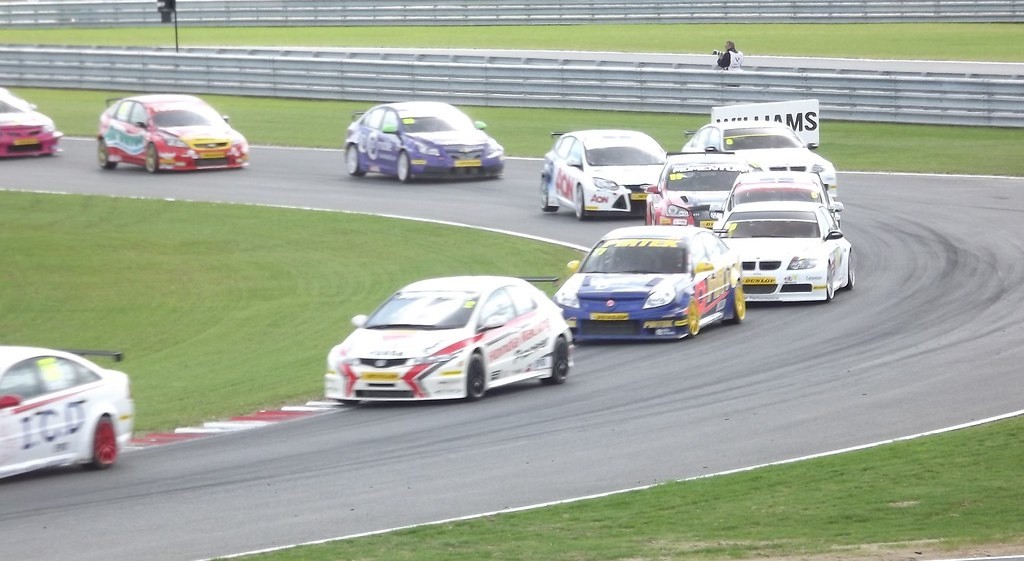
xmin=0 ymin=343 xmax=133 ymax=483
xmin=710 ymin=170 xmax=844 ymax=235
xmin=713 ymin=202 xmax=855 ymax=305
xmin=552 ymin=225 xmax=747 ymax=347
xmin=321 ymin=274 xmax=576 ymax=405
xmin=644 ymin=150 xmax=754 ymax=232
xmin=682 ymin=120 xmax=839 ymax=203
xmin=96 ymin=94 xmax=250 ymax=175
xmin=539 ymin=129 xmax=672 ymax=222
xmin=342 ymin=101 xmax=506 ymax=186
xmin=0 ymin=86 xmax=64 ymax=160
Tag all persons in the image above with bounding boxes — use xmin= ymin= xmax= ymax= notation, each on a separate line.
xmin=716 ymin=41 xmax=743 ymax=88
